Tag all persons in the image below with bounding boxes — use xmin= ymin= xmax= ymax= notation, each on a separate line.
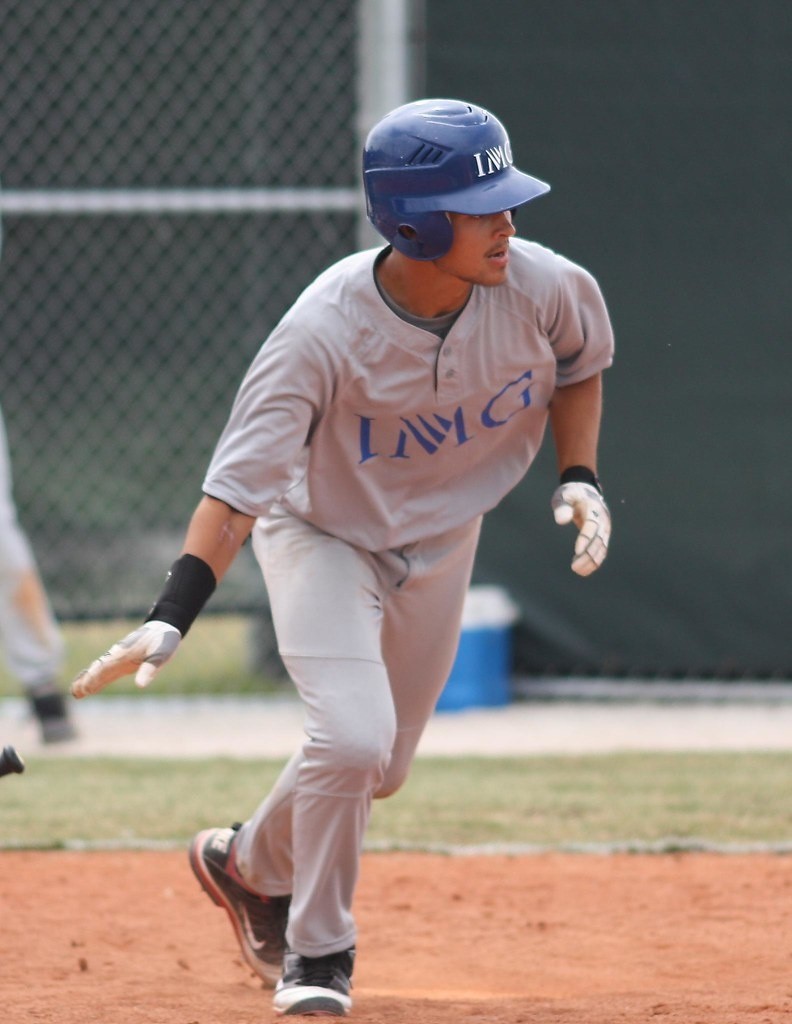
xmin=73 ymin=96 xmax=616 ymax=1019
xmin=0 ymin=409 xmax=80 ymax=777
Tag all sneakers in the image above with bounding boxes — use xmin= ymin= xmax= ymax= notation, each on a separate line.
xmin=270 ymin=944 xmax=356 ymax=1017
xmin=190 ymin=822 xmax=293 ymax=986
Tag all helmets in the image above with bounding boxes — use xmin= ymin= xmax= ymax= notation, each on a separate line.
xmin=362 ymin=98 xmax=553 ymax=261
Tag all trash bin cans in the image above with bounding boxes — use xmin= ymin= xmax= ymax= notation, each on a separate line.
xmin=433 ymin=587 xmax=524 ymax=715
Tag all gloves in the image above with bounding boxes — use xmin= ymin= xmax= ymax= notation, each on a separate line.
xmin=550 ymin=465 xmax=612 ymax=578
xmin=70 ymin=555 xmax=218 ymax=698
